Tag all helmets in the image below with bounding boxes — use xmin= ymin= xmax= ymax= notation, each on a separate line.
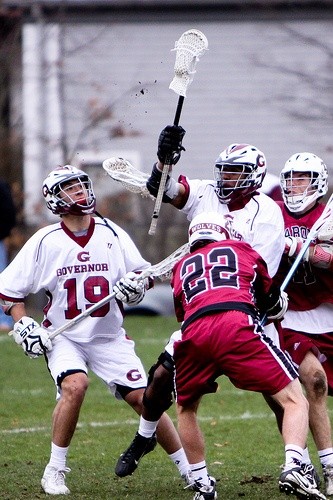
xmin=188 ymin=212 xmax=231 ymax=254
xmin=278 ymin=152 xmax=329 ymax=212
xmin=214 ymin=144 xmax=265 ymax=204
xmin=42 ymin=164 xmax=96 ymax=216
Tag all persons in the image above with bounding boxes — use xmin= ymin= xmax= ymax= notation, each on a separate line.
xmin=0 ymin=181 xmax=16 ymax=332
xmin=115 ymin=125 xmax=320 ymax=486
xmin=171 ymin=212 xmax=327 ymax=500
xmin=0 ymin=165 xmax=217 ymax=495
xmin=274 ymin=153 xmax=333 ymax=495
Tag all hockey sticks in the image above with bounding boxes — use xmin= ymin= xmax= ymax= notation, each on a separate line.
xmin=261 ymin=190 xmax=333 ymax=325
xmin=48 ymin=242 xmax=190 ymax=340
xmin=146 ymin=27 xmax=211 ymax=238
xmin=102 ymin=155 xmax=173 ymax=202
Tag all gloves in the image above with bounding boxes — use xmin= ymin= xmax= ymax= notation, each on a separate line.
xmin=7 ymin=316 xmax=53 ymax=359
xmin=284 ymin=236 xmax=308 ymax=262
xmin=113 ymin=272 xmax=145 ymax=306
xmin=267 ymin=291 xmax=289 ymax=319
xmin=156 ymin=125 xmax=186 ymax=164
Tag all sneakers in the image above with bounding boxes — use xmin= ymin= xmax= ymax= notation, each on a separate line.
xmin=193 ymin=476 xmax=218 ymax=500
xmin=321 ymin=463 xmax=333 ymax=495
xmin=40 ymin=464 xmax=71 ymax=495
xmin=300 ymin=463 xmax=322 ymax=491
xmin=278 ymin=464 xmax=327 ymax=500
xmin=182 ymin=470 xmax=196 ymax=490
xmin=115 ymin=431 xmax=157 ymax=477
